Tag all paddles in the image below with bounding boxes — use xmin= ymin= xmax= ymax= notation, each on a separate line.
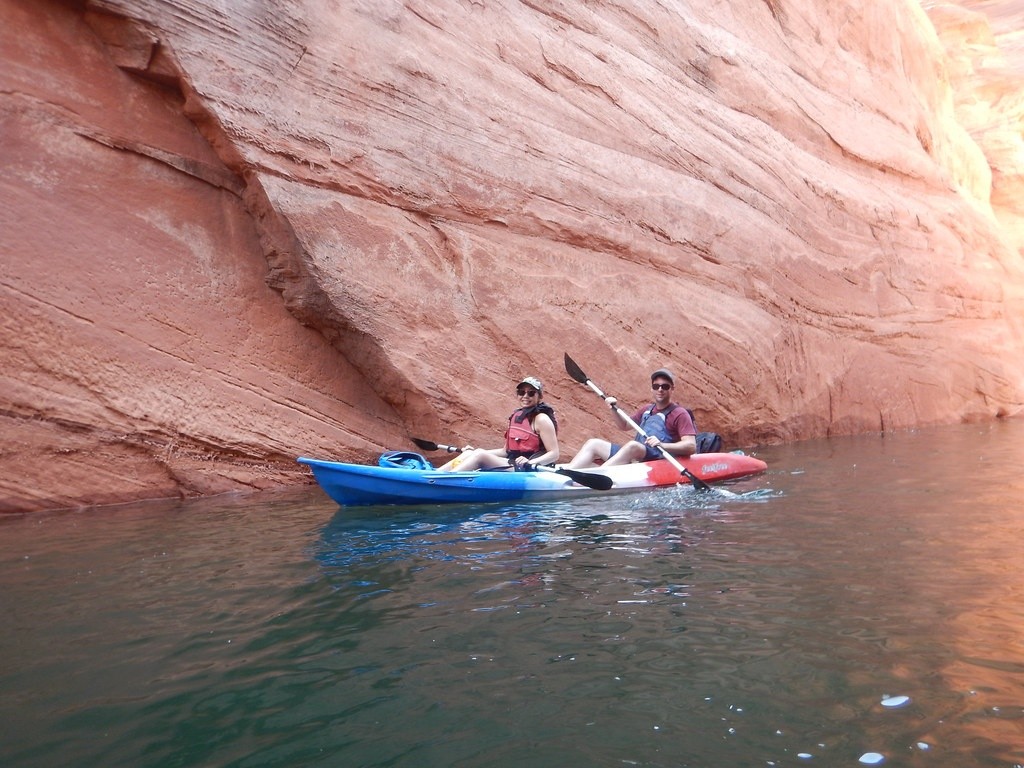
xmin=410 ymin=434 xmax=614 ymax=493
xmin=563 ymin=351 xmax=710 ymax=491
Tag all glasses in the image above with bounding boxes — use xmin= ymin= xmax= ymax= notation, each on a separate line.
xmin=517 ymin=389 xmax=536 ymax=397
xmin=652 ymin=384 xmax=670 ymax=390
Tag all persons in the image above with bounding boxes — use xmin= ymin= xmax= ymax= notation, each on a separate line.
xmin=436 ymin=376 xmax=559 ymax=472
xmin=566 ymin=369 xmax=697 ymax=467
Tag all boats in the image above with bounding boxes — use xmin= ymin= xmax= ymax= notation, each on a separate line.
xmin=297 ymin=452 xmax=767 ymax=508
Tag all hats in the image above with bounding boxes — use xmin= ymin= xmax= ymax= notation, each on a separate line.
xmin=516 ymin=377 xmax=543 ymax=395
xmin=651 ymin=369 xmax=674 ymax=384
